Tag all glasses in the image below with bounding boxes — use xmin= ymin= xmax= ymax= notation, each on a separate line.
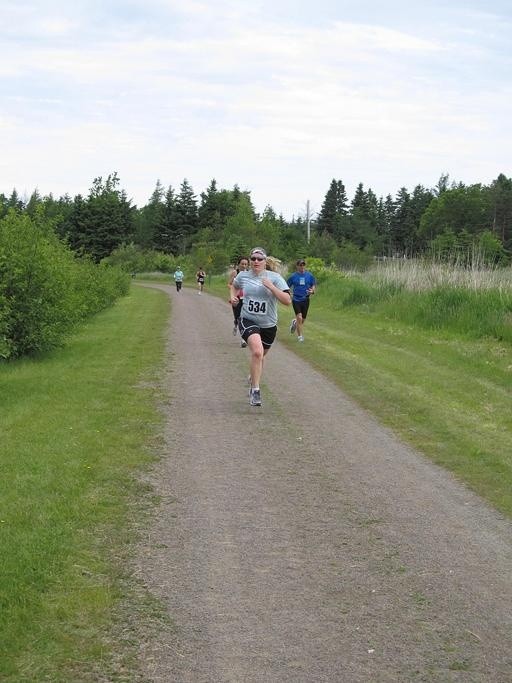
xmin=251 ymin=256 xmax=264 ymax=262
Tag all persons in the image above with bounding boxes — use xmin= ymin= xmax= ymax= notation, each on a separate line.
xmin=227 ymin=256 xmax=250 ymax=348
xmin=230 ymin=246 xmax=293 ymax=406
xmin=174 ymin=265 xmax=184 ymax=294
xmin=195 ymin=266 xmax=207 ymax=296
xmin=286 ymin=258 xmax=317 ymax=343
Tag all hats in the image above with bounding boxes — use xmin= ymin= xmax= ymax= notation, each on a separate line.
xmin=251 ymin=246 xmax=266 ymax=259
xmin=297 ymin=259 xmax=305 ymax=266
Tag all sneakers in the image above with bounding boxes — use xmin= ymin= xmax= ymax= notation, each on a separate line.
xmin=247 ymin=377 xmax=262 ymax=406
xmin=291 ymin=320 xmax=303 ymax=342
xmin=233 ymin=327 xmax=247 ymax=347
